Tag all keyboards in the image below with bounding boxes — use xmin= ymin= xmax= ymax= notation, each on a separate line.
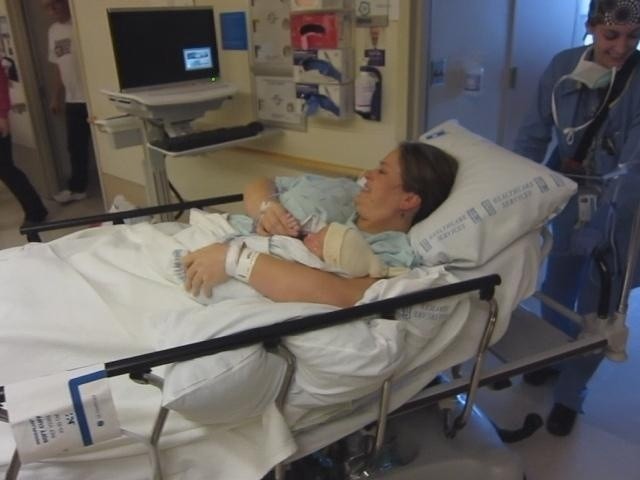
xmin=149 ymin=126 xmax=257 ymax=152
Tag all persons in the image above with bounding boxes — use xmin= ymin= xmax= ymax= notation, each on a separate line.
xmin=513 ymin=0 xmax=640 ymax=437
xmin=270 ymin=213 xmax=411 ymax=281
xmin=0 ymin=38 xmax=48 ymax=226
xmin=182 ymin=140 xmax=458 ymax=309
xmin=45 ymin=0 xmax=86 ymax=203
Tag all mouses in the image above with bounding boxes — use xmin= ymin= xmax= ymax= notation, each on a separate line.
xmin=248 ymin=122 xmax=263 ymax=131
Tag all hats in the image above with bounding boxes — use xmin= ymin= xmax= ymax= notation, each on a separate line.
xmin=323 ymin=222 xmax=409 ymax=279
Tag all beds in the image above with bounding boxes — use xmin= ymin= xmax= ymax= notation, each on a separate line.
xmin=1 ymin=115 xmax=637 ymax=480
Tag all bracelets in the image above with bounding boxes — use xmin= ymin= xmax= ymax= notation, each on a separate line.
xmin=225 ymin=239 xmax=261 ymax=283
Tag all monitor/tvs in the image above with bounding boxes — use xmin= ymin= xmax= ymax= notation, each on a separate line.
xmin=106 ymin=6 xmax=220 ymax=94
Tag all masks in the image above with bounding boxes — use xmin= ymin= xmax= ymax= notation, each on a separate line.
xmin=568 ymin=60 xmax=612 ymax=90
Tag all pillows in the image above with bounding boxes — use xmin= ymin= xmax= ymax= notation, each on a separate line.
xmin=377 ymin=115 xmax=580 ymax=273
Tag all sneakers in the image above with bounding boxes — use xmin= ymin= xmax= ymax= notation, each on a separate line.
xmin=547 ymin=402 xmax=577 ymax=437
xmin=524 ymin=367 xmax=560 ymax=383
xmin=53 ymin=189 xmax=86 ymax=203
